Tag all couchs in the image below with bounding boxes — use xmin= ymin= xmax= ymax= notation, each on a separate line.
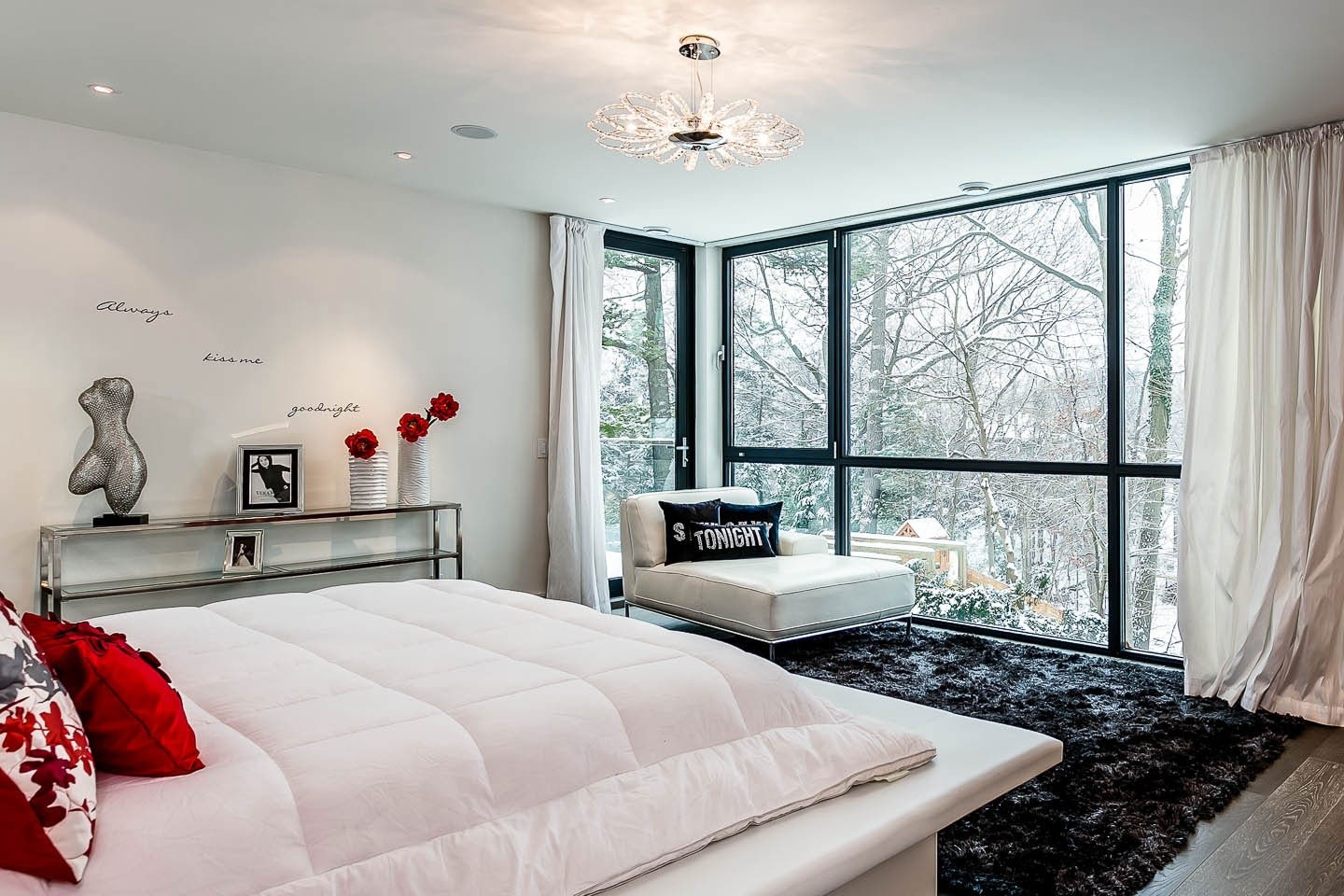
xmin=620 ymin=487 xmax=915 ymax=662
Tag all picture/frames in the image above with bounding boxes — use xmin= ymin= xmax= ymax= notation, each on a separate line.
xmin=236 ymin=443 xmax=304 ymax=516
xmin=222 ymin=529 xmax=265 ymax=576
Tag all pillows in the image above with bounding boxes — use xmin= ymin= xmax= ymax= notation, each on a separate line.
xmin=719 ymin=501 xmax=784 ymax=550
xmin=658 ymin=498 xmax=721 ymax=566
xmin=0 ymin=590 xmax=96 ymax=884
xmin=690 ymin=521 xmax=775 ymax=562
xmin=22 ymin=611 xmax=205 ymax=778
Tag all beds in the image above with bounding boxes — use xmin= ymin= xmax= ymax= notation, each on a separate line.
xmin=0 ymin=579 xmax=1063 ymax=896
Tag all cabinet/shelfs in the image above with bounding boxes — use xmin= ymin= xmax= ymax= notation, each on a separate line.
xmin=39 ymin=500 xmax=463 ymax=622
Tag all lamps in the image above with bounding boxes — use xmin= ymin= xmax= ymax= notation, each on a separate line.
xmin=587 ymin=32 xmax=803 ymax=174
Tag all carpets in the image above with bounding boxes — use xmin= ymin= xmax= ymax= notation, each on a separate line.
xmin=776 ymin=621 xmax=1306 ymax=896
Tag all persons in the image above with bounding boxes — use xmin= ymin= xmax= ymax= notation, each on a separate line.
xmin=251 ymin=455 xmax=291 ymax=503
xmin=233 ymin=541 xmax=252 ymax=566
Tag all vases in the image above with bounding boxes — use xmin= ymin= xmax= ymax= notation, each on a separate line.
xmin=348 ymin=449 xmax=389 ymax=510
xmin=397 ymin=431 xmax=430 ymax=506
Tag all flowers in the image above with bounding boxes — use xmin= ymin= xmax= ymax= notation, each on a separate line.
xmin=344 ymin=429 xmax=378 ymax=458
xmin=397 ymin=392 xmax=460 ymax=442
